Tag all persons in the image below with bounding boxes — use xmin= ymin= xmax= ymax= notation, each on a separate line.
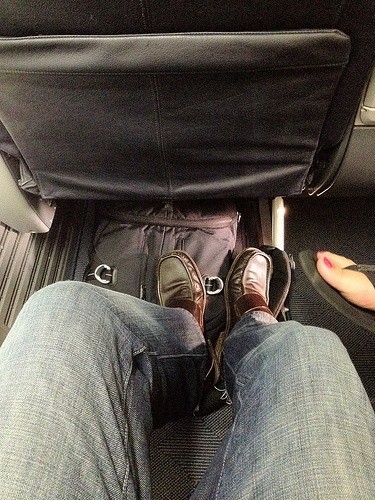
xmin=0 ymin=245 xmax=375 ymax=500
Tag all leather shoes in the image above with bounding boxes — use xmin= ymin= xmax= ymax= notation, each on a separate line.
xmin=216 ymin=246 xmax=274 ymax=380
xmin=157 ymin=250 xmax=219 ymax=388
